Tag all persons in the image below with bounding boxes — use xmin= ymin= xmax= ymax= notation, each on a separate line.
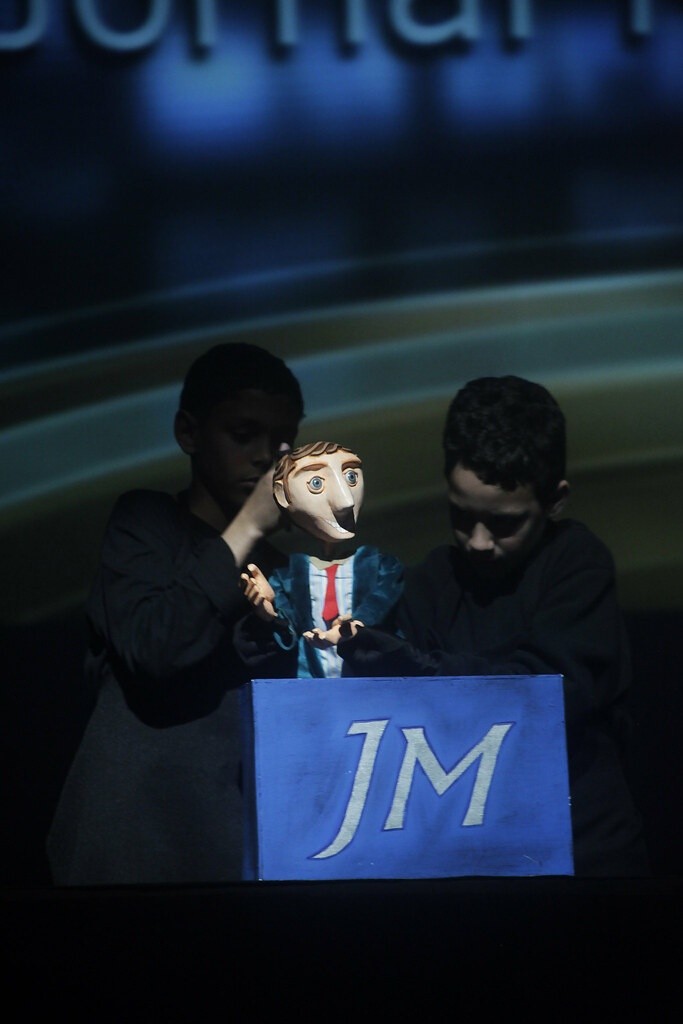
xmin=45 ymin=343 xmax=307 ymax=887
xmin=238 ymin=440 xmax=413 ymax=679
xmin=344 ymin=376 xmax=654 ymax=875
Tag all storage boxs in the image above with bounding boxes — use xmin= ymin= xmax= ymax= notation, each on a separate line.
xmin=237 ymin=667 xmax=574 ymax=878
